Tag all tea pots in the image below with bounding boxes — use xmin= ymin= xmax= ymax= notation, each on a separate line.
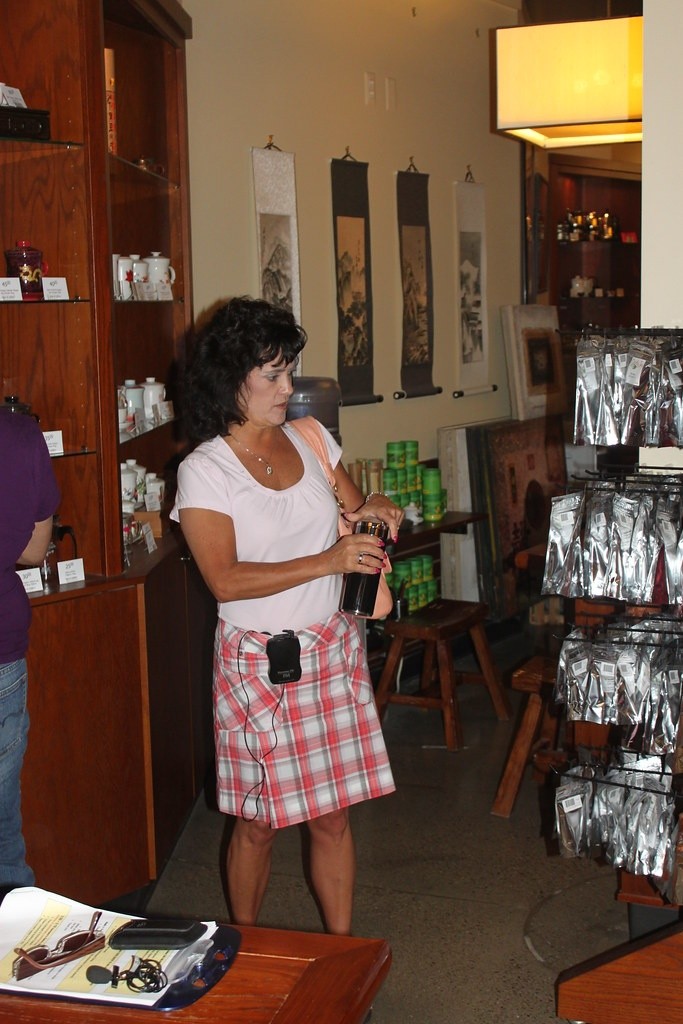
xmin=132 ymin=155 xmax=165 ymax=176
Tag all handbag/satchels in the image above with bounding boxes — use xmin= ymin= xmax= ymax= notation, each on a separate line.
xmin=288 ymin=415 xmax=392 ymax=618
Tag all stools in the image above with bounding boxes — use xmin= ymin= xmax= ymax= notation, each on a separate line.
xmin=490 ymin=655 xmax=561 ymax=817
xmin=375 ymin=598 xmax=510 ymax=751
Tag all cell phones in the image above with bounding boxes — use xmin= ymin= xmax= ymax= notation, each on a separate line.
xmin=121 ymin=919 xmax=197 ymax=935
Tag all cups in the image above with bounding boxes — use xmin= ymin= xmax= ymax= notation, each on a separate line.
xmin=119 ymin=463 xmax=135 ymax=504
xmin=123 ymin=380 xmax=144 ymax=421
xmin=5 ymin=240 xmax=47 ymax=300
xmin=145 ymin=252 xmax=174 ymax=298
xmin=118 ymin=258 xmax=133 ymax=300
xmin=143 ymin=377 xmax=165 ymax=417
xmin=146 ymin=474 xmax=165 ymax=502
xmin=127 ymin=460 xmax=146 ymax=506
xmin=130 ymin=255 xmax=148 ymax=298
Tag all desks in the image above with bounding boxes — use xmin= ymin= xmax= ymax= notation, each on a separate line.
xmin=0 ymin=923 xmax=393 ymax=1024
xmin=365 ymin=510 xmax=476 ymax=670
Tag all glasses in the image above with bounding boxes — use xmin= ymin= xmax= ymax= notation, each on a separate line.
xmin=12 ymin=912 xmax=105 ymax=981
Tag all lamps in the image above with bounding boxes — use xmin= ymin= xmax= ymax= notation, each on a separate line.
xmin=496 ymin=0 xmax=643 ymax=149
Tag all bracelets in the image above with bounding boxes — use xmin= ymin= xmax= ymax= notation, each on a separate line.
xmin=366 ymin=491 xmax=388 ymax=503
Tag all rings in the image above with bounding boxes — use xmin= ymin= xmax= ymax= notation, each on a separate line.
xmin=359 ymin=553 xmax=363 ymax=563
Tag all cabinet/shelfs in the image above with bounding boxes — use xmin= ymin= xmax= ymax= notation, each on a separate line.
xmin=549 ymin=163 xmax=640 ymax=410
xmin=0 ymin=0 xmax=194 ymax=905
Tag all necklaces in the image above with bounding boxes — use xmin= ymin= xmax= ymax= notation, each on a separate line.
xmin=231 ymin=426 xmax=278 ymax=474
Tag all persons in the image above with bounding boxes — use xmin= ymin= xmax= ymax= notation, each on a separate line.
xmin=169 ymin=295 xmax=406 ymax=935
xmin=0 ymin=406 xmax=59 ymax=895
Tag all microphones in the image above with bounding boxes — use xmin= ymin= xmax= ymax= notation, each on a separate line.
xmin=85 ymin=964 xmax=120 ymax=988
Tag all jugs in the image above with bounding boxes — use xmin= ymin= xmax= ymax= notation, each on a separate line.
xmin=40 ymin=515 xmax=77 ymax=581
xmin=0 ymin=396 xmax=39 ymax=422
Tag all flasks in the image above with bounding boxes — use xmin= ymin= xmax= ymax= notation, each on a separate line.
xmin=338 ymin=522 xmax=388 ymax=617
